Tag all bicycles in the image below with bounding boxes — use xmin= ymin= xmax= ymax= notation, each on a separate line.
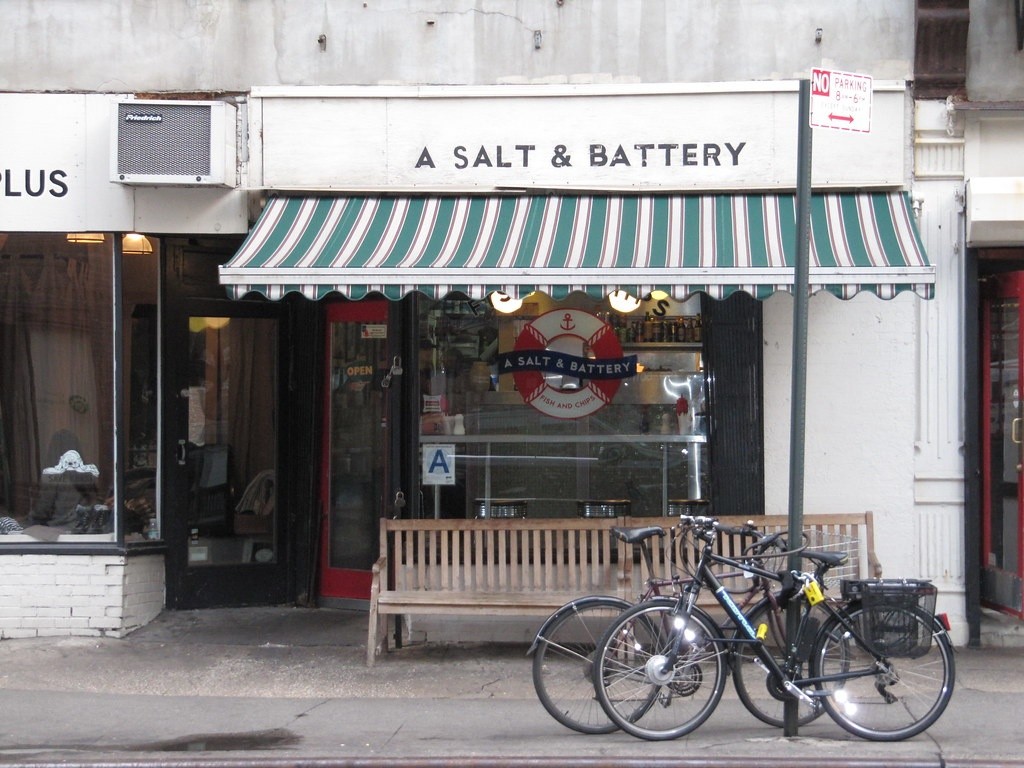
xmin=526 ymin=514 xmax=960 ymax=743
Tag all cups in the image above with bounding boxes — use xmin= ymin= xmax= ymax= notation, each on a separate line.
xmin=442 ymin=416 xmax=456 ymax=435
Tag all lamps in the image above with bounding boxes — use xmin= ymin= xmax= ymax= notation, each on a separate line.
xmin=121 ymin=233 xmax=153 ymax=254
xmin=66 ymin=232 xmax=104 ymax=244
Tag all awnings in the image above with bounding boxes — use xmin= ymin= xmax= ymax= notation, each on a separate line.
xmin=219 ymin=185 xmax=934 ymax=301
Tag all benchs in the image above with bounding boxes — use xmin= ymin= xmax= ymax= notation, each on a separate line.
xmin=364 ymin=515 xmax=877 ymax=667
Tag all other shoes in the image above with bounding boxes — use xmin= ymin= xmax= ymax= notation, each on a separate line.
xmin=0 ymin=516 xmax=23 ymax=534
xmin=90 ymin=509 xmax=113 ymax=533
xmin=71 ymin=506 xmax=91 ymax=533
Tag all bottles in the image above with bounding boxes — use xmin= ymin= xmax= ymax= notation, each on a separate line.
xmin=606 ymin=311 xmax=703 ymax=344
xmin=147 ymin=518 xmax=159 ymax=541
